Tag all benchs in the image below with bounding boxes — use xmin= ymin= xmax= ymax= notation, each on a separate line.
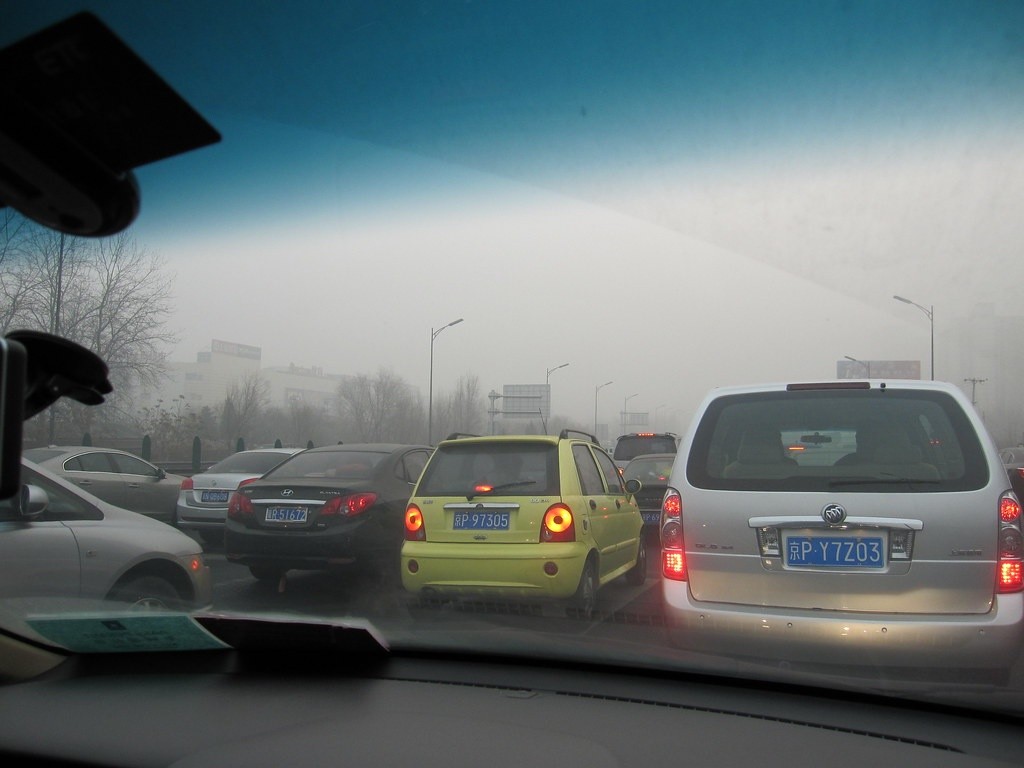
xmin=722 ymin=419 xmax=939 ymax=482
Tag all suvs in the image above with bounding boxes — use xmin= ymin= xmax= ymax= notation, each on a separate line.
xmin=401 ymin=429 xmax=649 ymax=620
xmin=612 ymin=380 xmax=1024 ymax=686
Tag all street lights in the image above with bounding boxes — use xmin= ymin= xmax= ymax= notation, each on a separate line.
xmin=546 ymin=363 xmax=569 ymax=433
xmin=428 ymin=319 xmax=464 ymax=445
xmin=595 ymin=382 xmax=614 ymax=438
xmin=845 ymin=356 xmax=872 ymax=379
xmin=893 ymin=295 xmax=934 ymax=381
xmin=624 ymin=394 xmax=638 ymax=434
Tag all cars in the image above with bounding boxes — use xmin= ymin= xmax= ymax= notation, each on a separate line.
xmin=177 ymin=447 xmax=304 ymax=545
xmin=0 ymin=456 xmax=215 ymax=614
xmin=224 ymin=443 xmax=435 ymax=585
xmin=22 ymin=444 xmax=187 ymax=525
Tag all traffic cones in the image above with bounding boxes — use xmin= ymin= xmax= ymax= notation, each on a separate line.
xmin=965 ymin=378 xmax=984 ymax=407
